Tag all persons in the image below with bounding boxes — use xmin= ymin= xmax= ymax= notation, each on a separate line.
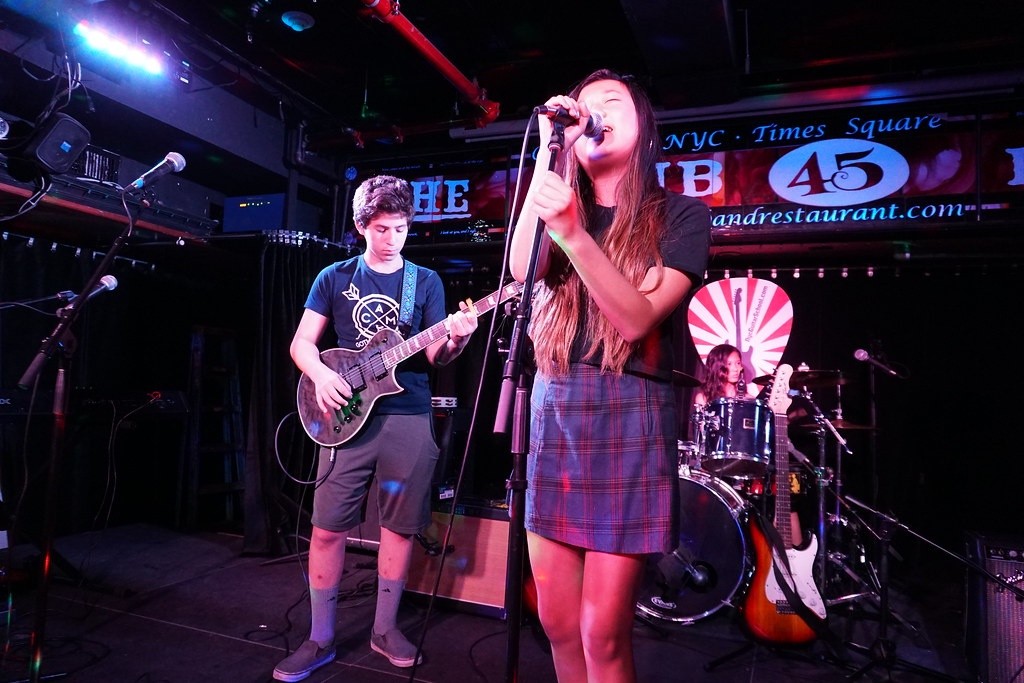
xmin=509 ymin=69 xmax=710 ymax=683
xmin=694 ymin=344 xmax=755 ymax=408
xmin=273 ymin=176 xmax=478 ymax=683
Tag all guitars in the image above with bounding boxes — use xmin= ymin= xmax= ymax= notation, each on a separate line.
xmin=294 ymin=273 xmax=538 ymax=448
xmin=743 ymin=363 xmax=828 ymax=643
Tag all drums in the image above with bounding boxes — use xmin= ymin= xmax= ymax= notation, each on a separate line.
xmin=677 ymin=395 xmax=776 ymax=482
xmin=633 ymin=468 xmax=757 ymax=626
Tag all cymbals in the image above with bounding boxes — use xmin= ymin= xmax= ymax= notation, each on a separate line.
xmin=800 ymin=418 xmax=874 ymax=433
xmin=751 ymin=363 xmax=853 ymax=390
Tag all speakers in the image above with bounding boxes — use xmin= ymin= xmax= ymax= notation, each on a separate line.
xmin=24 ymin=112 xmax=90 ymax=175
xmin=399 ymin=494 xmax=509 ymax=621
xmin=960 ymin=537 xmax=1024 ymax=683
xmin=345 ymin=473 xmax=381 ymax=552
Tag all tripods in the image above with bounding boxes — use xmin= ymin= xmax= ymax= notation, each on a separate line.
xmin=796 ymin=372 xmax=1024 ymax=683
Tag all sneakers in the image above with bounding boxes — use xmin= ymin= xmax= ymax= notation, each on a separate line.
xmin=273 ymin=634 xmax=336 ymax=683
xmin=370 ymin=626 xmax=422 ymax=667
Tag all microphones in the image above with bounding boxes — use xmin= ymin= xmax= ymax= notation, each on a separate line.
xmin=58 ymin=275 xmax=119 ymax=318
xmin=123 ymin=151 xmax=187 ymax=193
xmin=854 ymin=350 xmax=901 ymax=377
xmin=692 ymin=565 xmax=710 ymax=585
xmin=533 ymin=104 xmax=604 ymax=137
xmin=427 ymin=545 xmax=456 ymax=556
